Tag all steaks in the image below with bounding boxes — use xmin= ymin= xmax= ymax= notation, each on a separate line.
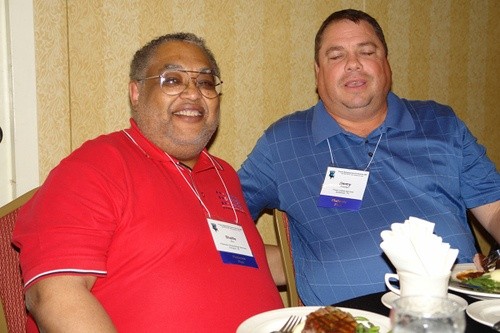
xmin=301 ymin=305 xmax=357 ymax=333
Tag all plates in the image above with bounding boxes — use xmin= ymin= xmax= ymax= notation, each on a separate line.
xmin=466 ymin=299 xmax=500 ymax=332
xmin=235 ymin=306 xmax=391 ymax=333
xmin=381 ymin=290 xmax=469 ymax=318
xmin=447 ymin=262 xmax=500 ymax=300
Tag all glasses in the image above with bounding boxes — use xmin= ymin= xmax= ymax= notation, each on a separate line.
xmin=137 ymin=70 xmax=224 ymax=99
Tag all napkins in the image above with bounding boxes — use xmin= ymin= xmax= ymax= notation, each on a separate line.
xmin=380 ymin=216 xmax=459 ymax=273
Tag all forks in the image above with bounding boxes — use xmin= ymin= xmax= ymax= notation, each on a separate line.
xmin=270 ymin=315 xmax=302 ymax=333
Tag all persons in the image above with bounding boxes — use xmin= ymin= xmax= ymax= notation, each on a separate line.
xmin=236 ymin=11 xmax=500 ymax=333
xmin=10 ymin=31 xmax=289 ymax=333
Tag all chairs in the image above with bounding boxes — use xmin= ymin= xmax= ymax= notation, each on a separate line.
xmin=0 ymin=185 xmax=41 ymax=333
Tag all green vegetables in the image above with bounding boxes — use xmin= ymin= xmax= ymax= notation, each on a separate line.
xmin=460 ymin=275 xmax=500 ymax=294
xmin=354 ymin=316 xmax=380 ymax=333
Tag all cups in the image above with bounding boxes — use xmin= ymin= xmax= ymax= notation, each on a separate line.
xmin=390 ymin=297 xmax=467 ymax=333
xmin=385 ymin=270 xmax=451 ymax=296
